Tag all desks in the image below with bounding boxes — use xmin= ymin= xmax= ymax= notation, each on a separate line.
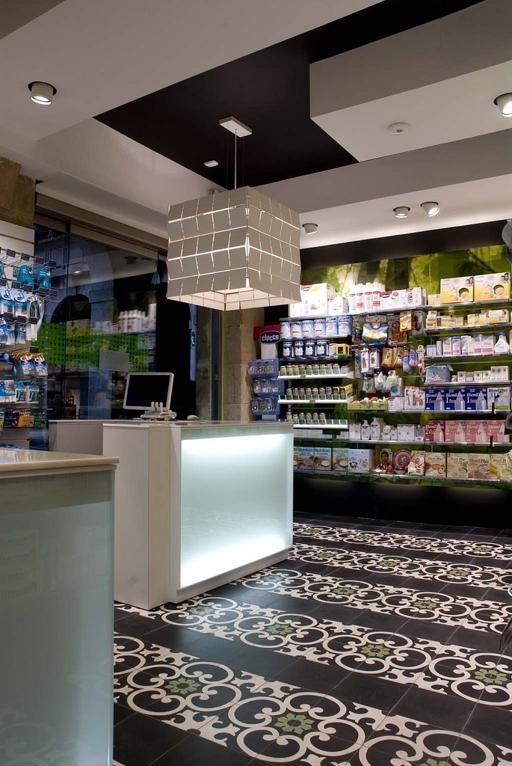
xmin=48 ymin=418 xmax=295 ymax=611
xmin=0 ymin=445 xmax=120 ymax=766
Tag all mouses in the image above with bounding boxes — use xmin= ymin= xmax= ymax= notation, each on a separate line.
xmin=186 ymin=414 xmax=199 ymax=420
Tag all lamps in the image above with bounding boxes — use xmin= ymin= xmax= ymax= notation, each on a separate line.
xmin=393 ymin=206 xmax=410 ymax=218
xmin=166 ymin=116 xmax=302 ymax=313
xmin=303 ymin=223 xmax=319 ymax=234
xmin=493 ymin=92 xmax=512 ymax=117
xmin=420 ymin=202 xmax=439 ymax=217
xmin=28 ymin=81 xmax=57 ymax=105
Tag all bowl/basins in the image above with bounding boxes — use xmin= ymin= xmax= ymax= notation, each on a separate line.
xmin=395 ymin=470 xmax=405 ymax=474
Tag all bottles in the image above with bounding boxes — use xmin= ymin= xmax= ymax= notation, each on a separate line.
xmin=455 ymin=422 xmax=465 ymax=443
xmin=434 ymin=391 xmax=445 ymax=410
xmin=433 ymin=422 xmax=445 ymax=443
xmin=116 ymin=308 xmax=146 ymax=332
xmin=494 ymin=332 xmax=511 ymax=353
xmin=475 ymin=422 xmax=488 ymax=443
xmin=454 ymin=391 xmax=466 ymax=410
xmin=348 ymin=281 xmax=388 ymax=313
xmin=476 ymin=390 xmax=487 ymax=409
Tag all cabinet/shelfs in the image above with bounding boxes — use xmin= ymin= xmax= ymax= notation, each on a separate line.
xmin=261 ymin=298 xmax=512 ymax=484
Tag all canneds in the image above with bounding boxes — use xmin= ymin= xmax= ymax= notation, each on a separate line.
xmin=283 ymin=341 xmax=327 ymax=356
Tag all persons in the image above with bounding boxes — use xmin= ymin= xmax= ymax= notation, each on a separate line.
xmin=380 ymin=451 xmax=390 ymax=470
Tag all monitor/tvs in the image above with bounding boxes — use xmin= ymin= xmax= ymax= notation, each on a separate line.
xmin=123 ymin=372 xmax=175 ymax=411
